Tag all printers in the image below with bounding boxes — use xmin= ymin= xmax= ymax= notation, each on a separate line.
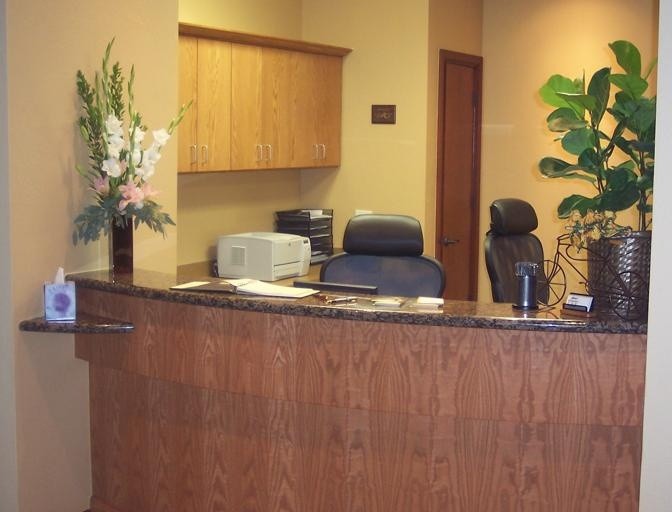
xmin=217 ymin=232 xmax=311 ymax=281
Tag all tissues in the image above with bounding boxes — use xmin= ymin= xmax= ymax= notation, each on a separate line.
xmin=42 ymin=266 xmax=77 ymax=322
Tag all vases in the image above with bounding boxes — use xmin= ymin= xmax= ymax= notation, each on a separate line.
xmin=108 ymin=214 xmax=134 ymax=275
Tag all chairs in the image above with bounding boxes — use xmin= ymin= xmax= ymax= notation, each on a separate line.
xmin=484 ymin=197 xmax=550 ymax=306
xmin=319 ymin=212 xmax=449 ymax=302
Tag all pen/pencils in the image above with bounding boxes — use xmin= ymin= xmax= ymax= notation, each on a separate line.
xmin=326 ymin=297 xmax=359 ymax=304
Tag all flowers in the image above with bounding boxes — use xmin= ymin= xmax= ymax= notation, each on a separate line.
xmin=65 ymin=35 xmax=194 ymax=245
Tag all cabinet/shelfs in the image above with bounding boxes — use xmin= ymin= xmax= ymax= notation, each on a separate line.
xmin=292 ymin=49 xmax=343 ymax=169
xmin=231 ymin=40 xmax=293 ymax=172
xmin=178 ymin=34 xmax=232 ymax=175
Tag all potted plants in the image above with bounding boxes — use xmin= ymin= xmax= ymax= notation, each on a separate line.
xmin=537 ymin=35 xmax=658 ymax=315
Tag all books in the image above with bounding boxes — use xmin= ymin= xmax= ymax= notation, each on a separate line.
xmin=169 ymin=277 xmax=320 ymax=300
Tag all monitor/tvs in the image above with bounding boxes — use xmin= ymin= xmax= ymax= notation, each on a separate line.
xmin=294 ymin=280 xmax=378 ymax=295
